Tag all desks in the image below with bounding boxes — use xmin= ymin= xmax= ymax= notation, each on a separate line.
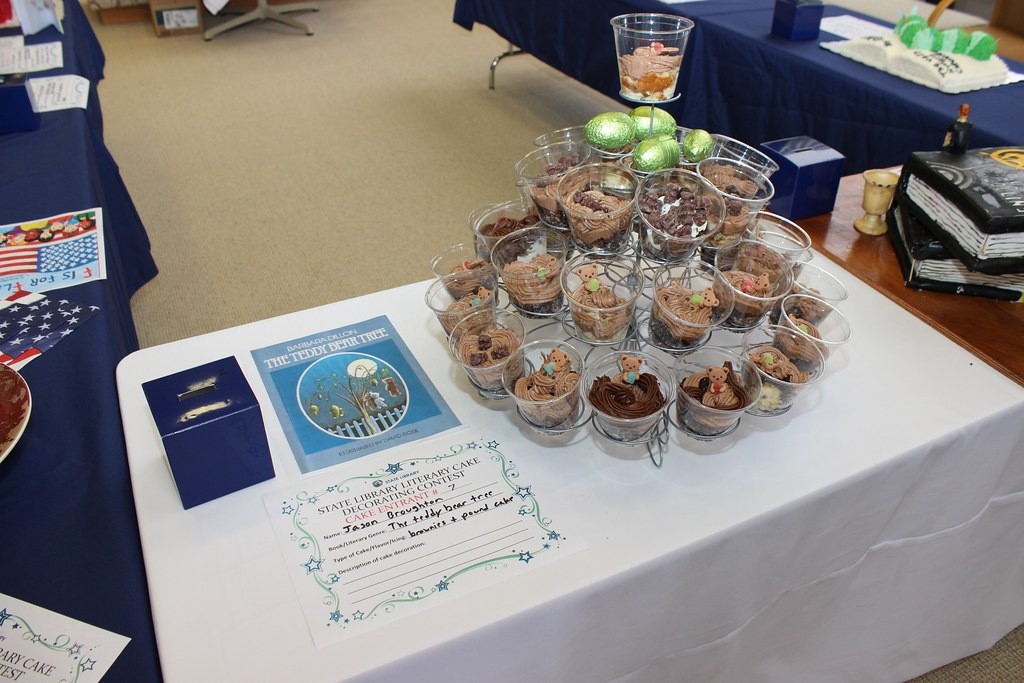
xmin=0 ymin=0 xmax=159 ymax=683
xmin=116 ymin=166 xmax=1024 ymax=683
xmin=454 ymin=1 xmax=1024 ymax=175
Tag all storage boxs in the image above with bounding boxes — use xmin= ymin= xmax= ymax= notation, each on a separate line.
xmin=149 ymin=0 xmax=204 ymax=37
xmin=887 ymin=145 xmax=1024 ymax=302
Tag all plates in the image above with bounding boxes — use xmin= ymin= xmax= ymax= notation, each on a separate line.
xmin=0 ymin=361 xmax=33 ymax=464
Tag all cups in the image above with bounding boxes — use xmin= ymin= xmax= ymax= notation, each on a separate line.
xmin=611 ymin=13 xmax=695 ymax=100
xmin=852 ymin=169 xmax=900 ymax=236
xmin=423 ymin=126 xmax=851 ymax=440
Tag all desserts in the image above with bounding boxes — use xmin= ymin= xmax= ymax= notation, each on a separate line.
xmin=610 ymin=13 xmax=695 ymax=102
xmin=423 ymin=126 xmax=853 ymax=450
